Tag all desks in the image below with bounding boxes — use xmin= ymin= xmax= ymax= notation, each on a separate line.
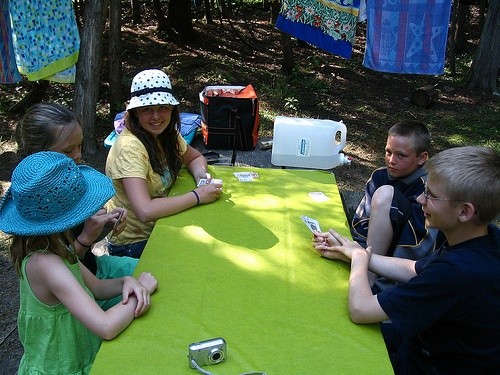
xmin=89 ymin=168 xmax=396 ymax=374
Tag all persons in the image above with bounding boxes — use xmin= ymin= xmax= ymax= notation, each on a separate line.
xmin=103 ymin=70 xmax=222 ymax=259
xmin=0 ymin=151 xmax=160 ymax=375
xmin=311 ymin=145 xmax=500 ymax=375
xmin=15 ymin=101 xmax=143 ymax=313
xmin=350 ymin=120 xmax=445 ymax=298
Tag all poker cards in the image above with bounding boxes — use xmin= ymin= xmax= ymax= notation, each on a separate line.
xmin=307 ymin=191 xmax=328 ymax=203
xmin=234 ymin=170 xmax=259 ymax=181
xmin=197 ymin=177 xmax=222 ymax=189
xmin=300 ymin=216 xmax=322 ymax=236
xmin=107 ymin=207 xmax=126 ymax=238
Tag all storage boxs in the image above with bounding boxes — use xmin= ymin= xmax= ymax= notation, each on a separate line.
xmin=198 ymin=84 xmax=260 ymax=152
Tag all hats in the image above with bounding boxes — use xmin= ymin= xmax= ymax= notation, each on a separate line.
xmin=125 ymin=69 xmax=180 ymax=111
xmin=0 ymin=150 xmax=116 ymax=236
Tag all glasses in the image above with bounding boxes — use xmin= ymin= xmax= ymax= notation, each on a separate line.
xmin=423 ymin=185 xmax=478 ymax=215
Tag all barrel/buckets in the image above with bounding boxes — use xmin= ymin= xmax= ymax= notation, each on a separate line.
xmin=271 ymin=116 xmax=347 ymax=169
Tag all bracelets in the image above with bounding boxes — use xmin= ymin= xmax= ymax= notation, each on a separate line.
xmin=191 ymin=190 xmax=200 ymax=205
xmin=74 ymin=237 xmax=92 ymax=248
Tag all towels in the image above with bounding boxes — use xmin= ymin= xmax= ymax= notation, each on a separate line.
xmin=362 ymin=0 xmax=452 ymax=74
xmin=275 ymin=0 xmax=367 ymax=59
xmin=1 ymin=0 xmax=80 ymax=84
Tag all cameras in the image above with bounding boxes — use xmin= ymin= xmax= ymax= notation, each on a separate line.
xmin=189 ymin=337 xmax=227 ymax=368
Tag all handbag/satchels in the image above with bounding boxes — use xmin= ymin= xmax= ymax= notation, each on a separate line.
xmin=199 ymin=84 xmax=259 ymax=151
